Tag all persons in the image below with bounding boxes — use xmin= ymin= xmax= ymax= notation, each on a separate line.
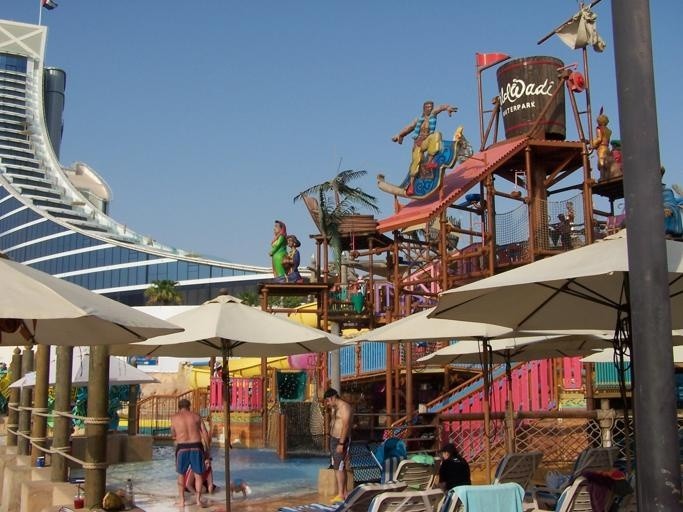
xmin=365 ymin=384 xmax=386 ymax=440
xmin=137 ymin=353 xmax=158 ymax=367
xmin=272 ymin=235 xmax=301 ymax=282
xmin=609 ymin=140 xmax=624 ymax=180
xmin=269 ymin=220 xmax=288 ymax=278
xmin=174 ymin=361 xmax=225 ymax=509
xmin=658 ymin=164 xmax=683 ymax=239
xmin=549 ymin=202 xmax=575 ymax=249
xmin=323 ymin=389 xmax=354 ymax=502
xmin=350 ymin=284 xmax=365 ymax=315
xmin=434 ymin=443 xmax=471 ymax=497
xmin=588 ymin=111 xmax=612 ymax=183
xmin=348 ymin=250 xmax=357 ymax=283
xmin=391 ymin=101 xmax=457 ymax=195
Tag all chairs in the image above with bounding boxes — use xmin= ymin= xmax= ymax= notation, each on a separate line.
xmin=272 ymin=446 xmax=639 ymax=511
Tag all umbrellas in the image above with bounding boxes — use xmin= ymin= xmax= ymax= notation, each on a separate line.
xmin=415 ymin=326 xmax=682 ymax=448
xmin=83 ymin=294 xmax=358 ymax=511
xmin=427 ymin=227 xmax=683 ymax=449
xmin=345 ymin=303 xmax=604 ymax=485
xmin=8 ymin=345 xmax=159 ymax=388
xmin=0 ymin=257 xmax=185 ymax=345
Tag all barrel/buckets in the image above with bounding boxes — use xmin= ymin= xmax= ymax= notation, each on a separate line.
xmin=74 ymin=496 xmax=84 ymax=509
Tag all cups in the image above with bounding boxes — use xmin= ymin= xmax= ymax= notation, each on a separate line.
xmin=72 ymin=495 xmax=85 ymax=508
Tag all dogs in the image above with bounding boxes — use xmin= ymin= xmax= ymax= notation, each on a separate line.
xmin=228 ymin=479 xmax=253 ymax=499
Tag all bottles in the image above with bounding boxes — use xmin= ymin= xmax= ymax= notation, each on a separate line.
xmin=125 ymin=478 xmax=133 ymax=508
